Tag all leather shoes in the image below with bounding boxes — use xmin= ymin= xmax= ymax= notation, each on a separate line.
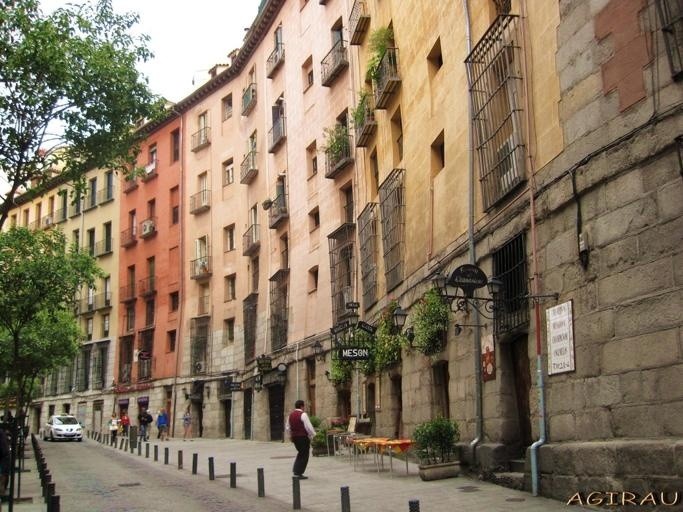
xmin=293 ymin=475 xmax=307 ymax=479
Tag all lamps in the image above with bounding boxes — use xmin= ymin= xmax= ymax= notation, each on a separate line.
xmin=392 ymin=306 xmax=408 ymax=337
xmin=311 ymin=340 xmax=325 ymax=363
xmin=431 ymin=271 xmax=503 ymax=300
xmin=346 ymin=310 xmax=360 ymax=327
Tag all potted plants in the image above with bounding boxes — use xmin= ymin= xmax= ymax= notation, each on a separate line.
xmin=412 ymin=410 xmax=462 ymax=481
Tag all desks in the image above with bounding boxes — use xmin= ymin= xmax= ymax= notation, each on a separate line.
xmin=326 ymin=429 xmax=416 ymax=479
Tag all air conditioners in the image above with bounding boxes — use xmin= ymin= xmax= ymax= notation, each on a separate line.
xmin=195 ymin=361 xmax=205 ymax=373
xmin=141 ymin=219 xmax=153 ymax=238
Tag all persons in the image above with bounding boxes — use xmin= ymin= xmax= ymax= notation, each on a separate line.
xmin=283 ymin=398 xmax=318 ymax=481
xmin=105 ymin=405 xmax=170 ymax=443
xmin=180 ymin=403 xmax=194 ymax=442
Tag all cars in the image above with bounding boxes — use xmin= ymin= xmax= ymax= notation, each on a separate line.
xmin=43 ymin=414 xmax=85 ymax=442
